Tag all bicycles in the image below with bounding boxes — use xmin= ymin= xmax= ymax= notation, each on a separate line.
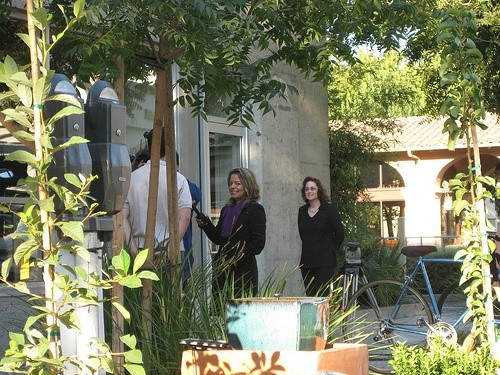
xmin=341 ymin=233 xmax=500 ymax=375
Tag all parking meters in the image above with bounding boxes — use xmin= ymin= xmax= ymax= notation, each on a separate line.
xmin=42 ymin=73 xmax=133 ymax=375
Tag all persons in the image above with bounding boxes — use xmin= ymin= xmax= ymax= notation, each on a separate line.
xmin=115 ymin=127 xmax=202 ymax=294
xmin=195 ymin=168 xmax=266 ymax=298
xmin=493 ymin=253 xmax=500 ymax=286
xmin=296 ymin=177 xmax=344 ymax=297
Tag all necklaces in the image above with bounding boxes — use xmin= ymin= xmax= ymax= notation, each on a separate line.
xmin=309 ymin=206 xmax=320 ymax=213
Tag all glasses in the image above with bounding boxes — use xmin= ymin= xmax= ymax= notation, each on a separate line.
xmin=304 ymin=187 xmax=319 ymax=192
